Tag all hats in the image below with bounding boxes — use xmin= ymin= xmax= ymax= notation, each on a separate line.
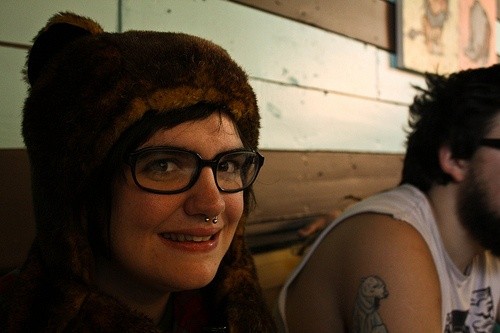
xmin=4 ymin=10 xmax=270 ymax=332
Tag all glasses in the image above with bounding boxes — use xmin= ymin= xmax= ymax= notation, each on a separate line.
xmin=113 ymin=145 xmax=264 ymax=194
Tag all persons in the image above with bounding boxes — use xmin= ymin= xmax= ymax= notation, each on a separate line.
xmin=262 ymin=63 xmax=500 ymax=333
xmin=292 ymin=184 xmax=403 ymax=254
xmin=0 ymin=12 xmax=280 ymax=333
